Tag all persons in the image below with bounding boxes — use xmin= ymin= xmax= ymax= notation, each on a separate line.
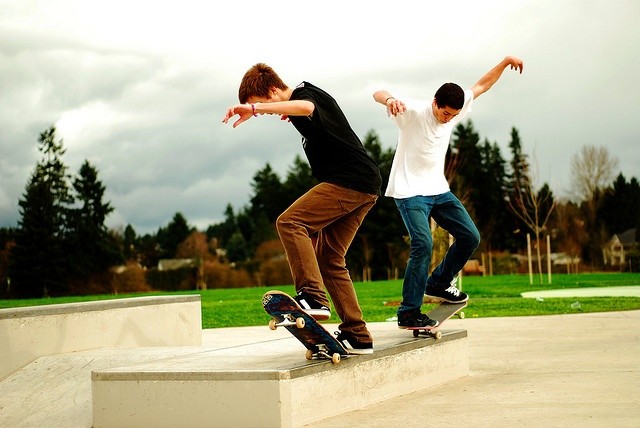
xmin=221 ymin=63 xmax=381 ymax=357
xmin=373 ymin=55 xmax=524 ymax=329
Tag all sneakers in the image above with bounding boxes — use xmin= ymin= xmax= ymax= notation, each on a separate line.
xmin=333 ymin=331 xmax=374 ymax=354
xmin=293 ymin=292 xmax=331 ymax=320
xmin=423 ymin=285 xmax=469 ymax=304
xmin=397 ymin=311 xmax=439 ymax=329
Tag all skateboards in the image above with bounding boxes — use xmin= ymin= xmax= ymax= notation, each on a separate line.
xmin=407 ymin=301 xmax=467 ymax=340
xmin=261 ymin=290 xmax=349 ymax=364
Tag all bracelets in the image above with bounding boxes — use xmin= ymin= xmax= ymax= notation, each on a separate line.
xmin=251 ymin=103 xmax=258 ymax=117
xmin=385 ymin=97 xmax=395 ymax=104
